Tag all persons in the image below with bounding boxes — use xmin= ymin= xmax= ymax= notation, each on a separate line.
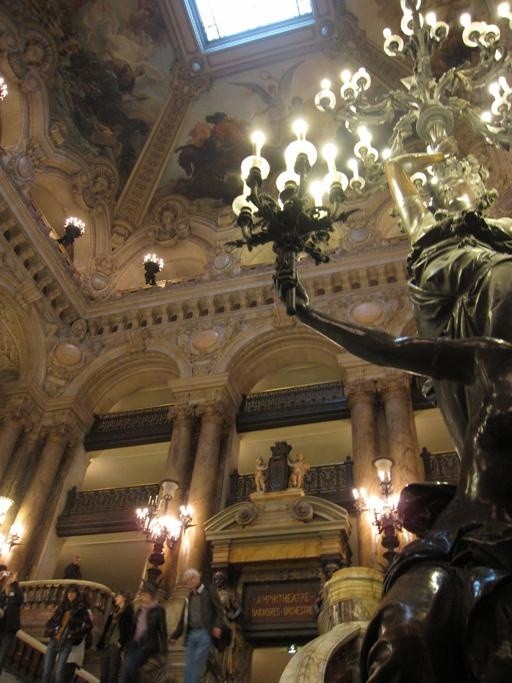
xmin=383 ymin=137 xmax=512 ymax=462
xmin=212 ymin=571 xmax=242 ymax=623
xmin=256 ymin=455 xmax=269 ymax=493
xmin=313 ymin=564 xmax=338 ymax=618
xmin=274 ymin=266 xmax=512 ymax=682
xmin=0 ymin=553 xmax=224 ymax=683
xmin=288 ymin=453 xmax=310 ymax=489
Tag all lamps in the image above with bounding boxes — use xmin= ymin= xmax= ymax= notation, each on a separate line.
xmin=350 ymin=455 xmax=412 ymax=564
xmin=54 ymin=212 xmax=89 ymax=250
xmin=1 ymin=493 xmax=28 ymax=560
xmin=224 ymin=113 xmax=380 ymax=317
xmin=129 ymin=475 xmax=199 ymax=596
xmin=311 ymin=0 xmax=511 ymax=217
xmin=135 ymin=250 xmax=167 ymax=287
xmin=0 ymin=75 xmax=10 ymax=106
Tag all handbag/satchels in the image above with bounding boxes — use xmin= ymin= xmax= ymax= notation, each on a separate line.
xmin=213 ymin=624 xmax=233 ymax=653
xmin=66 ymin=639 xmax=86 ymax=668
